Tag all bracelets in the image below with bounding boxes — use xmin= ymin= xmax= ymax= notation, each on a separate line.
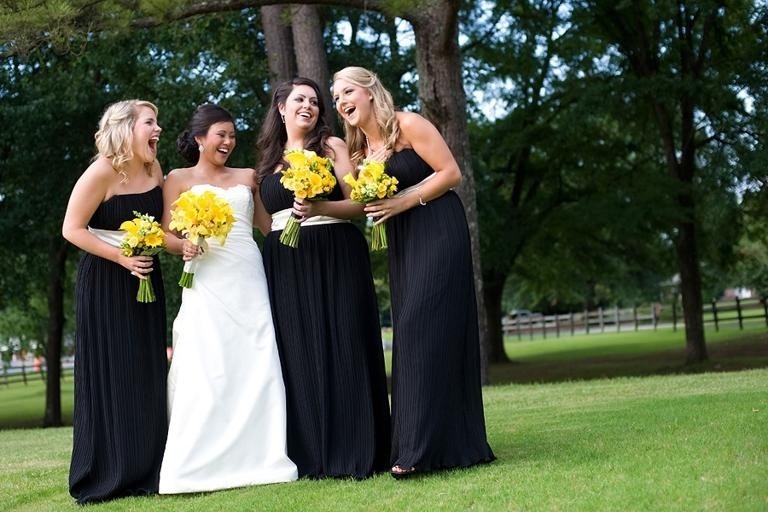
xmin=417 ymin=186 xmax=429 ymax=210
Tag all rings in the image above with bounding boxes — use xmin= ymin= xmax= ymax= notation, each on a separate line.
xmin=382 ymin=211 xmax=386 ymax=216
xmin=184 ymin=248 xmax=187 ymax=251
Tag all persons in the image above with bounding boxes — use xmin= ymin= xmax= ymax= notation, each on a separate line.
xmin=257 ymin=78 xmax=395 ymax=481
xmin=61 ymin=97 xmax=167 ymax=506
xmin=159 ymin=101 xmax=298 ymax=495
xmin=330 ymin=66 xmax=499 ymax=479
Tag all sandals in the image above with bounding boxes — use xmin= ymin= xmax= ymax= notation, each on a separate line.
xmin=392 ymin=465 xmax=417 ymax=477
